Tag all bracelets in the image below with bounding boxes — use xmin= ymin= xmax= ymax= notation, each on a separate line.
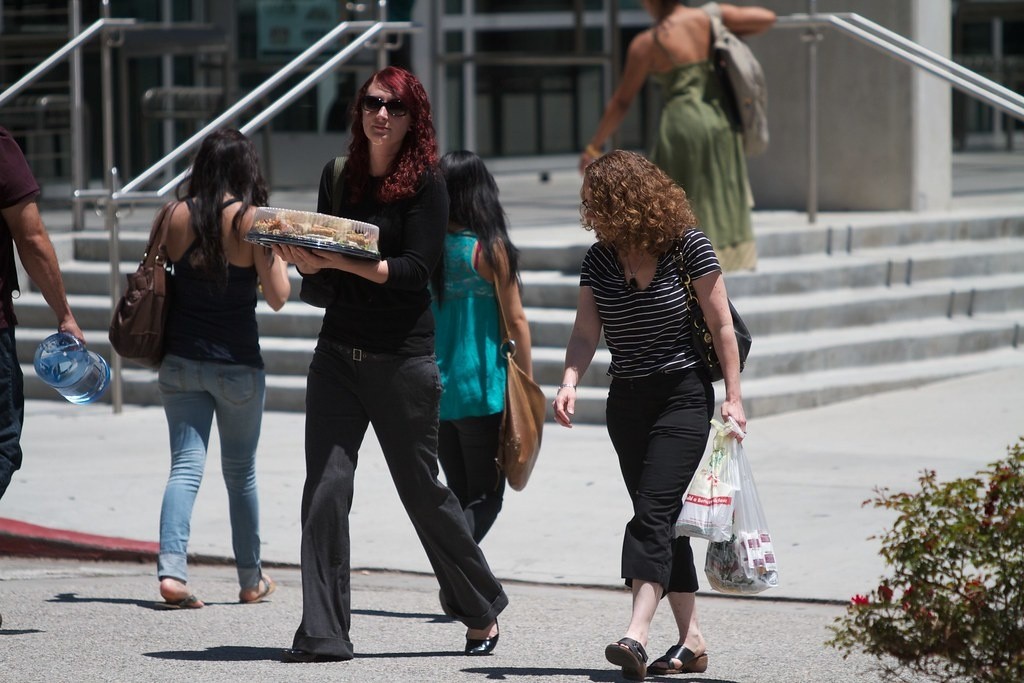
xmin=584 ymin=144 xmax=601 ymax=159
xmin=556 ymin=384 xmax=577 ymax=394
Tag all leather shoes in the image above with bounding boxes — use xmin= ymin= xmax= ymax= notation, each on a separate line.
xmin=465 ymin=617 xmax=499 ymax=656
xmin=281 ymin=647 xmax=316 ymax=661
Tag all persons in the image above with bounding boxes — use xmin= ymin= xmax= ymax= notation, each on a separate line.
xmin=267 ymin=70 xmax=510 ymax=661
xmin=141 ymin=128 xmax=291 ymax=611
xmin=0 ymin=126 xmax=87 ymax=502
xmin=551 ymin=149 xmax=748 ymax=679
xmin=426 ymin=149 xmax=535 ymax=616
xmin=578 ymin=0 xmax=778 ymax=275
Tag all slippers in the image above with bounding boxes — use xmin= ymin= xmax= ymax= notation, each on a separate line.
xmin=238 ymin=576 xmax=275 ymax=604
xmin=155 ymin=593 xmax=206 ymax=610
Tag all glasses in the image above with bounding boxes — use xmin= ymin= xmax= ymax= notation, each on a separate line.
xmin=361 ymin=96 xmax=409 ymax=117
xmin=581 ymin=199 xmax=587 ymax=213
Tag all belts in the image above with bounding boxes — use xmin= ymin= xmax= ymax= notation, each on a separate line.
xmin=330 ymin=342 xmax=402 ymax=361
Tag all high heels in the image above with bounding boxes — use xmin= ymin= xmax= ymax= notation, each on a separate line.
xmin=647 ymin=645 xmax=708 ymax=674
xmin=605 ymin=637 xmax=648 ymax=681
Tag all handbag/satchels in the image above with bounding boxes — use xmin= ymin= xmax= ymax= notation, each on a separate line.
xmin=675 ymin=420 xmax=736 ymax=542
xmin=110 ymin=201 xmax=180 ymax=370
xmin=705 ymin=416 xmax=778 ymax=594
xmin=498 ymin=353 xmax=545 ymax=491
xmin=673 ymin=235 xmax=752 ymax=382
xmin=702 ymin=2 xmax=768 ymax=153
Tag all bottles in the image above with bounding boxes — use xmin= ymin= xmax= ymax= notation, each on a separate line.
xmin=33 ymin=332 xmax=110 ymax=405
